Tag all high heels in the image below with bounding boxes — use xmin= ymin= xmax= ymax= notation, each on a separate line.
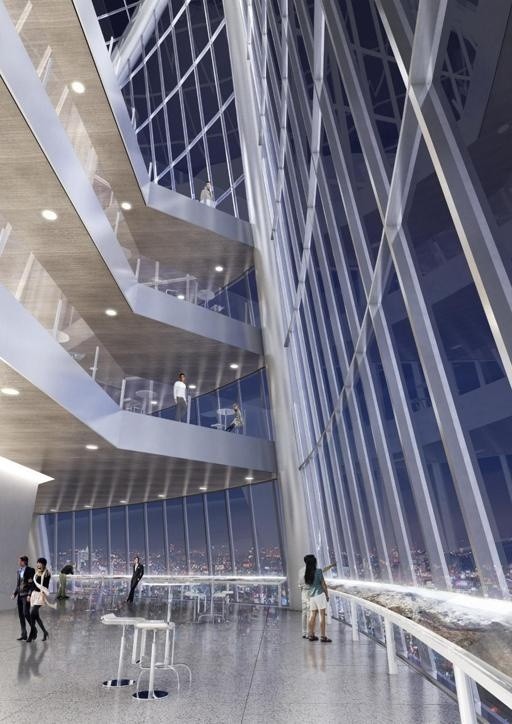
xmin=41 ymin=630 xmax=51 ymax=642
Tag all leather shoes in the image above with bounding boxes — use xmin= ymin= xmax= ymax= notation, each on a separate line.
xmin=26 ymin=630 xmax=38 ymax=642
xmin=16 ymin=635 xmax=28 ymax=641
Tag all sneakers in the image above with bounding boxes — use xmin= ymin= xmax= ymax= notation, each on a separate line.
xmin=56 ymin=595 xmax=71 ymax=599
xmin=302 ymin=635 xmax=333 ymax=642
